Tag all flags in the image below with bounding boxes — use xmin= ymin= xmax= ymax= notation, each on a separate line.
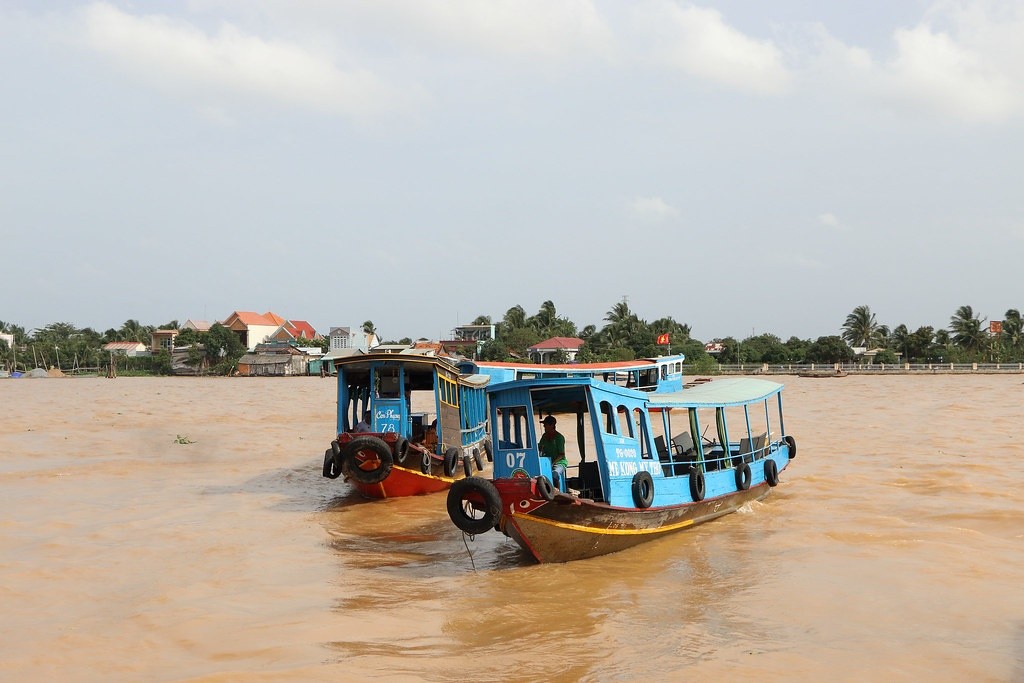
xmin=657 ymin=333 xmax=669 ymax=345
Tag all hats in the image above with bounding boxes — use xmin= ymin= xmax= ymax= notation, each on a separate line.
xmin=540 ymin=416 xmax=556 ymax=424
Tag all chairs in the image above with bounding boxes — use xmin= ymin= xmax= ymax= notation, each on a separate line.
xmin=654 ymin=435 xmax=669 ymax=460
xmin=594 ymin=461 xmax=603 ymax=502
xmin=577 ymin=461 xmax=601 ymax=502
xmin=754 ymin=432 xmax=768 ymax=460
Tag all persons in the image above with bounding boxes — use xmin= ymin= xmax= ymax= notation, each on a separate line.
xmin=353 ymin=410 xmax=371 ymax=433
xmin=539 ymin=416 xmax=568 ymax=489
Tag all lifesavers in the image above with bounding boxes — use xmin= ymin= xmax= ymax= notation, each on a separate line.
xmin=785 ymin=435 xmax=796 ymax=459
xmin=631 ymin=470 xmax=655 ymax=508
xmin=735 ymin=463 xmax=752 ymax=491
xmin=421 ymin=450 xmax=431 ymax=475
xmin=536 ymin=475 xmax=555 ymax=501
xmin=463 ymin=455 xmax=472 ymax=478
xmin=392 ymin=436 xmax=410 ymax=463
xmin=764 ymin=459 xmax=779 ymax=487
xmin=443 ymin=447 xmax=459 ymax=478
xmin=689 ymin=468 xmax=705 ymax=502
xmin=341 ymin=434 xmax=394 ymax=484
xmin=472 ymin=448 xmax=484 ymax=471
xmin=484 ymin=439 xmax=495 ymax=463
xmin=446 ymin=475 xmax=504 ymax=535
xmin=322 ymin=439 xmax=343 ymax=480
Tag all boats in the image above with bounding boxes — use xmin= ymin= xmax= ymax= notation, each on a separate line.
xmin=472 ymin=353 xmax=685 ymax=394
xmin=447 ymin=377 xmax=796 ymax=564
xmin=797 ymin=370 xmax=848 ymax=377
xmin=322 ymin=352 xmax=516 ymax=498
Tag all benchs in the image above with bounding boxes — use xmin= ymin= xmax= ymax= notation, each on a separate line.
xmin=671 ymin=431 xmax=713 ymax=474
xmin=733 ymin=436 xmax=759 ymax=466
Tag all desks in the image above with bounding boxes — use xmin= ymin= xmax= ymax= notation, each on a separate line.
xmin=712 ymin=446 xmax=740 ymax=470
